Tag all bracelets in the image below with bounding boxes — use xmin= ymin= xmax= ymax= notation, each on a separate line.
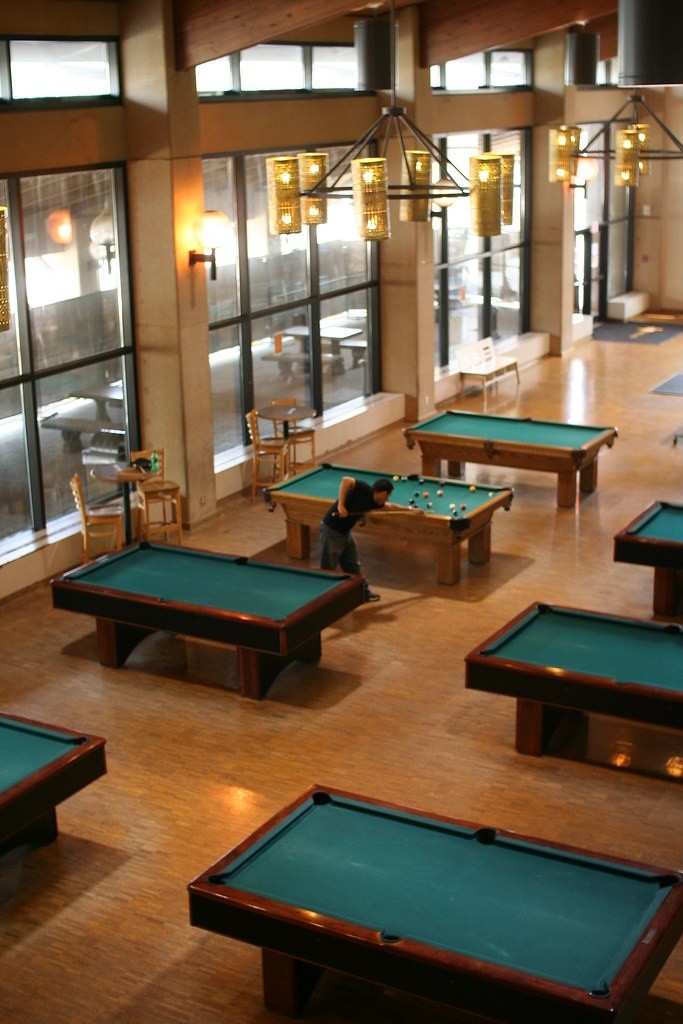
xmin=409 ymin=506 xmax=411 ymax=510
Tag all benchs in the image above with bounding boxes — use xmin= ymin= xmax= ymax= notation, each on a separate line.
xmin=454 ymin=338 xmax=523 ymax=402
xmin=259 ymin=339 xmax=366 ymax=365
xmin=45 ymin=413 xmax=125 ymax=457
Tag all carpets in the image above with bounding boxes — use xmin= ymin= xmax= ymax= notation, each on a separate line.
xmin=649 ymin=373 xmax=683 ymax=397
xmin=592 ymin=319 xmax=683 ymax=346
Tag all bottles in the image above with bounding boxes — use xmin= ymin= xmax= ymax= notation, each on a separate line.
xmin=151 ymin=450 xmax=159 ymax=471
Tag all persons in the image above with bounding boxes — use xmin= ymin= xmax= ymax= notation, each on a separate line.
xmin=320 ymin=477 xmax=424 ymax=602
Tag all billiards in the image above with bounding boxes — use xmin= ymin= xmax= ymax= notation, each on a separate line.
xmin=452 ymin=510 xmax=457 ymax=514
xmin=437 ymin=490 xmax=443 ymax=496
xmin=449 ymin=503 xmax=455 ymax=510
xmin=461 ymin=504 xmax=466 ymax=509
xmin=439 ymin=481 xmax=445 ymax=486
xmin=414 ymin=491 xmax=419 ymax=497
xmin=489 ymin=492 xmax=494 ymax=497
xmin=427 ymin=502 xmax=432 ymax=508
xmin=414 ymin=504 xmax=418 ymax=508
xmin=409 ymin=498 xmax=414 ymax=504
xmin=393 ymin=476 xmax=399 ymax=481
xmin=423 ymin=492 xmax=429 ymax=498
xmin=401 ymin=476 xmax=408 ymax=482
xmin=418 ymin=479 xmax=425 ymax=484
xmin=470 ymin=486 xmax=475 ymax=492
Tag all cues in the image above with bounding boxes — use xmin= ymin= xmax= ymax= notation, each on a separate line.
xmin=331 ymin=510 xmax=435 ymax=516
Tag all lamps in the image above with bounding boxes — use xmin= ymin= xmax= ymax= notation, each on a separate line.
xmin=564 ymin=22 xmax=599 ymax=87
xmin=90 ymin=197 xmax=118 ymax=275
xmin=617 ymin=0 xmax=683 ymax=87
xmin=265 ymin=5 xmax=515 ymax=241
xmin=547 ymin=82 xmax=682 ymax=184
xmin=187 ymin=209 xmax=231 ymax=282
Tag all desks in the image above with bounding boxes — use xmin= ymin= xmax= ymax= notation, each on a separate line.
xmin=89 ymin=463 xmax=162 ymax=542
xmin=267 ymin=464 xmax=514 ymax=583
xmin=464 ymin=600 xmax=683 ymax=757
xmin=406 ymin=406 xmax=617 ymax=506
xmin=187 ymin=784 xmax=683 ymax=1024
xmin=283 ymin=325 xmax=360 ymax=377
xmin=71 ymin=384 xmax=123 ymax=455
xmin=259 ymin=406 xmax=318 ymax=477
xmin=0 ymin=711 xmax=107 ymax=860
xmin=614 ymin=499 xmax=683 ymax=616
xmin=53 ymin=546 xmax=367 ymax=700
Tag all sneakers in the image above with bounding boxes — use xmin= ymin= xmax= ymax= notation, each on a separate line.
xmin=367 ymin=592 xmax=380 ymax=601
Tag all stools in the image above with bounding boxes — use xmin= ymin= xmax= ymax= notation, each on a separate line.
xmin=272 ymin=398 xmax=317 ymax=471
xmin=130 ymin=448 xmax=184 ymax=546
xmin=246 ymin=411 xmax=293 ymax=499
xmin=68 ymin=474 xmax=122 ymax=563
